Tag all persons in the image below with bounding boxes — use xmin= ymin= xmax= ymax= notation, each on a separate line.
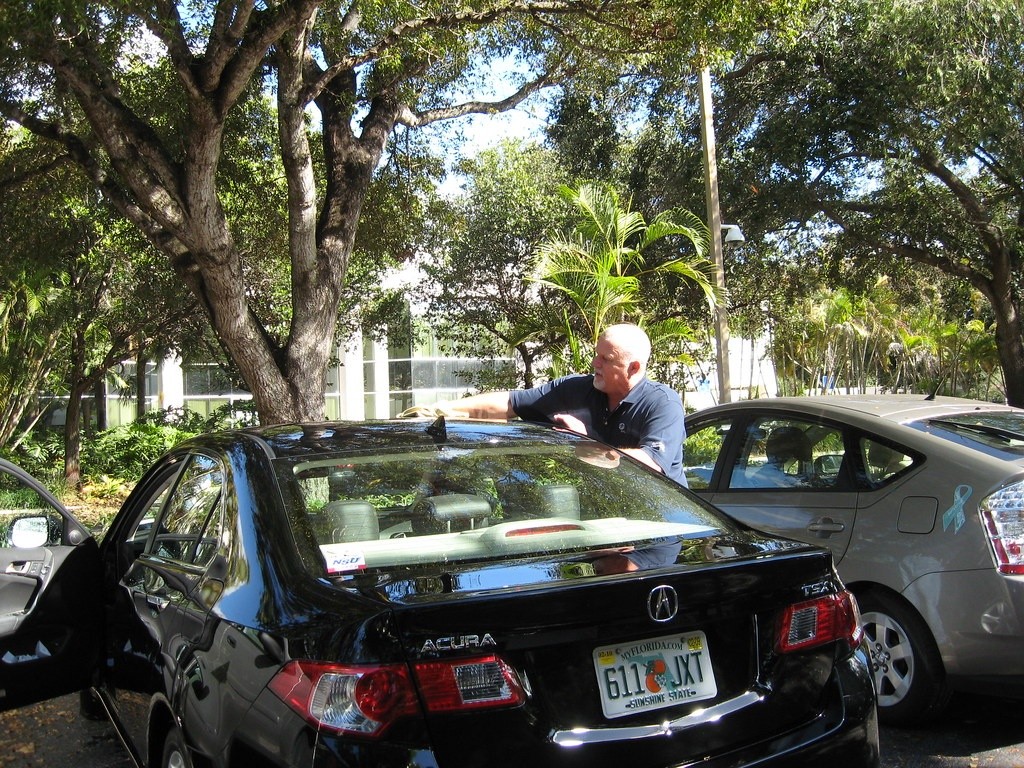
xmin=397 ymin=321 xmax=690 ymax=490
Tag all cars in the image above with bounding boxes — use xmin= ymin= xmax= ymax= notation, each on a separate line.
xmin=684 ymin=393 xmax=1024 ymax=727
xmin=2 ymin=414 xmax=881 ymax=768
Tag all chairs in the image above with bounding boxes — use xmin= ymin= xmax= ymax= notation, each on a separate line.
xmin=868 ymin=442 xmax=906 ymax=474
xmin=409 ymin=493 xmax=492 ymax=536
xmin=309 ymin=499 xmax=383 ymax=547
xmin=747 ymin=426 xmax=814 ymax=487
xmin=510 ymin=481 xmax=583 ymax=521
